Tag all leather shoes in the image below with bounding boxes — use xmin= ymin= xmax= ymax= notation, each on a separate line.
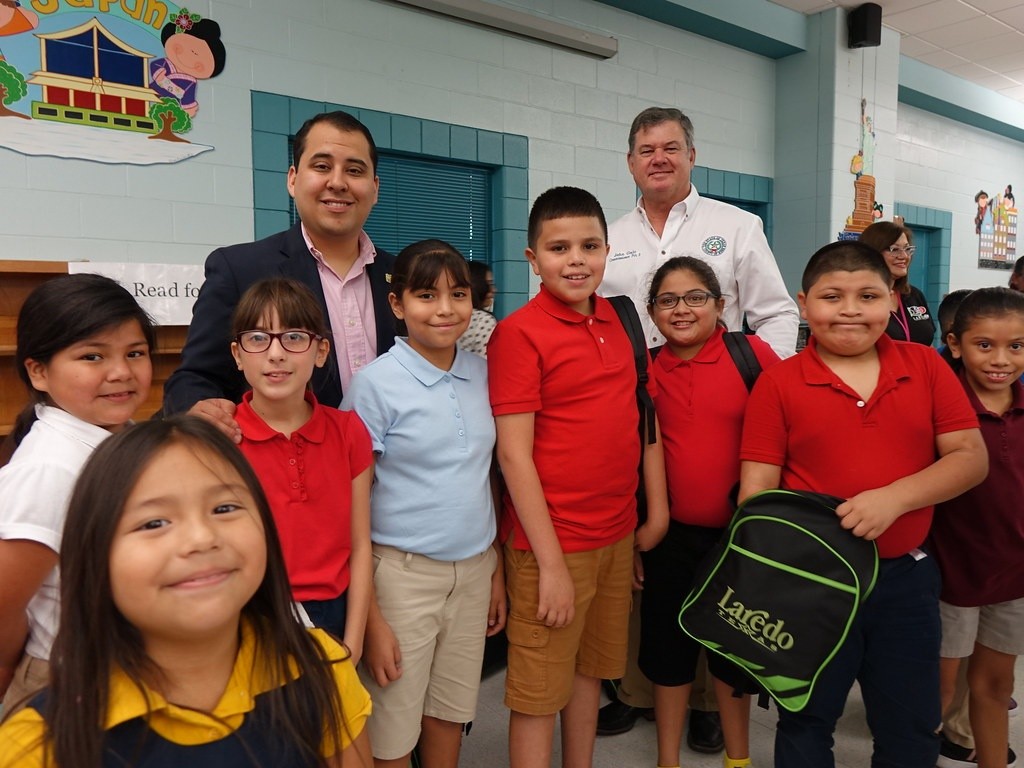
xmin=688 ymin=709 xmax=724 ymax=754
xmin=596 ymin=699 xmax=656 ymax=736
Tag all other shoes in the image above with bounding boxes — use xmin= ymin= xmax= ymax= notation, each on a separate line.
xmin=1008 ymin=699 xmax=1018 ymax=717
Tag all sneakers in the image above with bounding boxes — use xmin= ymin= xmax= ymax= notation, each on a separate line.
xmin=936 ymin=730 xmax=1018 ymax=768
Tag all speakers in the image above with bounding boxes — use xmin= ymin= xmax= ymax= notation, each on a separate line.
xmin=851 ymin=3 xmax=882 ymax=48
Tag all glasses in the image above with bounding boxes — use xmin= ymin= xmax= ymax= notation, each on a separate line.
xmin=234 ymin=329 xmax=324 ymax=353
xmin=652 ymin=289 xmax=719 ymax=310
xmin=885 ymin=244 xmax=916 ymax=256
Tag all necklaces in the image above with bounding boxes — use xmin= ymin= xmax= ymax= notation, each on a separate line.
xmin=891 ymin=291 xmax=910 ymax=342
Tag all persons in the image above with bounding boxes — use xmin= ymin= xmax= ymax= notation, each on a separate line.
xmin=0 ymin=107 xmax=799 ymax=768
xmin=737 ymin=221 xmax=1024 ymax=768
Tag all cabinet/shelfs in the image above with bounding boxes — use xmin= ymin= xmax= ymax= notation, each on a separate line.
xmin=0 ymin=260 xmax=207 ymax=459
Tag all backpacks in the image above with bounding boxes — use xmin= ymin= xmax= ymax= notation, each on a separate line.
xmin=678 ymin=488 xmax=879 ymax=717
